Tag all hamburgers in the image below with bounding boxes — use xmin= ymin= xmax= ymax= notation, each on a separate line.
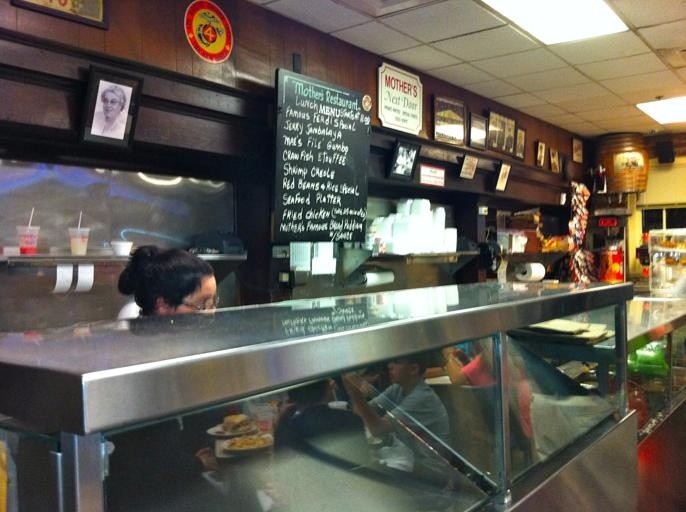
xmin=223 ymin=414 xmax=251 ymax=433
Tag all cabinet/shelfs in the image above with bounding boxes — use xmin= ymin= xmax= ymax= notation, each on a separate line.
xmin=0 ymin=277 xmax=684 ymax=510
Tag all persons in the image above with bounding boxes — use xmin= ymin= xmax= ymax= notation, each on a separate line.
xmin=103 ymin=245 xmax=263 ymax=512
xmin=91 ymin=85 xmax=127 ymax=140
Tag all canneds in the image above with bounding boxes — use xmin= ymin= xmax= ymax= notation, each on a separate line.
xmin=599 ymin=246 xmax=624 ymax=284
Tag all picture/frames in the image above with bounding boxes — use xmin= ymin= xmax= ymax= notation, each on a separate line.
xmin=75 ymin=60 xmax=145 ymax=153
xmin=483 ymin=107 xmax=515 ymax=158
xmin=466 ymin=111 xmax=487 ymax=150
xmin=431 ymin=92 xmax=467 ymax=148
xmin=514 ymin=126 xmax=527 ymax=162
xmin=385 ymin=135 xmax=422 ymax=184
xmin=535 ymin=136 xmax=583 ymax=173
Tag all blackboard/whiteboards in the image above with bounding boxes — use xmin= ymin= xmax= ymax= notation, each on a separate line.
xmin=273 ymin=69 xmax=372 ymax=242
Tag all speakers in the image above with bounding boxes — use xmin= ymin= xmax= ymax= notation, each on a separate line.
xmin=657 ymin=140 xmax=675 ymax=164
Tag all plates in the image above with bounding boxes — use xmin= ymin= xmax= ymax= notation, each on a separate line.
xmin=206 ymin=424 xmax=258 ymax=437
xmin=222 ymin=436 xmax=273 ymax=453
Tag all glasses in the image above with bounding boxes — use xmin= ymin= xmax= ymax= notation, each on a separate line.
xmin=176 ymin=298 xmax=220 ymax=312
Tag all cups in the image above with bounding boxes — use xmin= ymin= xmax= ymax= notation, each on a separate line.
xmin=111 ymin=241 xmax=133 ymax=256
xmin=15 ymin=225 xmax=40 ymax=254
xmin=328 ymin=400 xmax=349 ymax=410
xmin=68 ymin=227 xmax=89 ymax=258
xmin=252 ymin=400 xmax=278 ymax=435
